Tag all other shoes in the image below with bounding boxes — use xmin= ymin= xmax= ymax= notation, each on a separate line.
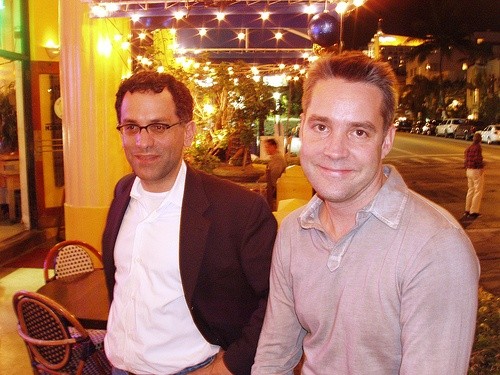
xmin=465 ymin=210 xmax=483 ymax=218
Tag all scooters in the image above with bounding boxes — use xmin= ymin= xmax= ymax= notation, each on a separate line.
xmin=410 ymin=117 xmax=436 ymax=135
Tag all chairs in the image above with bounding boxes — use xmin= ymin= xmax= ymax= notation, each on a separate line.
xmin=13 ymin=241 xmax=113 ymax=375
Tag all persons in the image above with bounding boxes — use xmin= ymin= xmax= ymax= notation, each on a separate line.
xmin=257 ymin=139 xmax=285 ymax=196
xmin=101 ymin=70 xmax=279 ymax=375
xmin=251 ymin=50 xmax=482 ymax=375
xmin=225 ymin=110 xmax=248 ymax=166
xmin=464 ymin=133 xmax=486 ymax=218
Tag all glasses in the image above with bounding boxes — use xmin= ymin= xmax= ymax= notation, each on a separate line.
xmin=116 ymin=121 xmax=185 ymax=138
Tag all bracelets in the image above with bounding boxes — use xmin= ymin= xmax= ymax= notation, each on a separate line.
xmin=230 ymin=158 xmax=234 ymax=159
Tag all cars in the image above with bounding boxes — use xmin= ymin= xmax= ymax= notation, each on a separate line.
xmin=435 ymin=118 xmax=466 ymax=138
xmin=475 ymin=124 xmax=500 ymax=144
xmin=393 ymin=117 xmax=412 ymax=133
xmin=454 ymin=119 xmax=486 ymax=141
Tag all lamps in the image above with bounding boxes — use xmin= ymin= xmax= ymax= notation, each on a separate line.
xmin=45 ymin=48 xmax=59 ymax=59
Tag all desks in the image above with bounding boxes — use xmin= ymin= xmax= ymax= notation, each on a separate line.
xmin=33 ymin=267 xmax=111 ymax=329
